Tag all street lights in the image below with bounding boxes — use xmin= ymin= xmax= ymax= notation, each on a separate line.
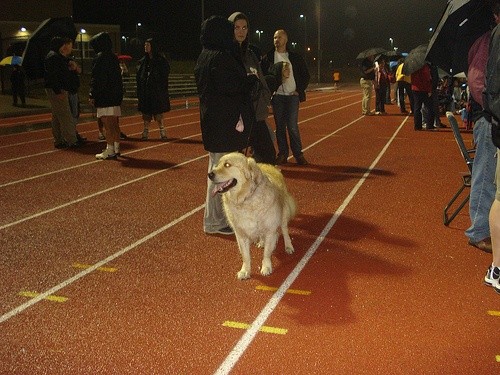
xmin=255 ymin=29 xmax=264 ymax=42
xmin=122 ymin=36 xmax=127 ymax=55
xmin=300 ymin=14 xmax=306 ymax=64
xmin=79 ymin=28 xmax=86 ymax=74
xmin=136 ymin=22 xmax=141 ymax=48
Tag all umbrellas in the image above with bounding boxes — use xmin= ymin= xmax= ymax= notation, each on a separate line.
xmin=355 ymin=48 xmax=385 ymax=60
xmin=19 ymin=17 xmax=78 ymax=78
xmin=423 ymin=0 xmax=500 ymax=78
xmin=374 ymin=50 xmax=404 ymax=63
xmin=401 ymin=43 xmax=428 ymax=76
xmin=0 ymin=54 xmax=23 ymax=67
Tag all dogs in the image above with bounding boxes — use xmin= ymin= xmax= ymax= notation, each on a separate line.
xmin=207 ymin=152 xmax=296 ymax=280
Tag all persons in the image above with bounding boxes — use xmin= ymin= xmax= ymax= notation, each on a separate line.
xmin=228 ymin=12 xmax=290 ymax=165
xmin=40 ymin=31 xmax=127 ymax=160
xmin=136 ymin=38 xmax=170 ymax=142
xmin=194 ymin=15 xmax=261 ymax=235
xmin=10 ymin=64 xmax=26 ymax=107
xmin=260 ymin=29 xmax=311 ymax=166
xmin=333 ymin=68 xmax=340 ymax=92
xmin=359 ymin=0 xmax=500 ymax=295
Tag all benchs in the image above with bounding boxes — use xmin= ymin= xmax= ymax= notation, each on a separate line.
xmin=122 ymin=72 xmax=198 ymax=98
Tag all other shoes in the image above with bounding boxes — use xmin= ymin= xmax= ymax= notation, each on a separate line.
xmin=363 ymin=111 xmax=373 ymax=116
xmin=400 ymin=108 xmax=408 ymax=114
xmin=375 ymin=110 xmax=386 ymax=116
xmin=417 ymin=121 xmax=446 ymax=130
xmin=159 ymin=130 xmax=167 ymax=140
xmin=120 ymin=132 xmax=127 ymax=140
xmin=70 ymin=141 xmax=85 ymax=148
xmin=296 ymin=152 xmax=309 ymax=165
xmin=468 ymin=237 xmax=493 ymax=254
xmin=142 ymin=132 xmax=150 ymax=140
xmin=98 ymin=132 xmax=106 ymax=141
xmin=277 ymin=153 xmax=288 ymax=163
xmin=77 ymin=134 xmax=88 ymax=141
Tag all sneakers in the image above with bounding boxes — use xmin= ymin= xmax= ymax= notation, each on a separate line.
xmin=96 ymin=149 xmax=116 ymax=159
xmin=103 ymin=146 xmax=121 ymax=157
xmin=483 ymin=263 xmax=500 ymax=292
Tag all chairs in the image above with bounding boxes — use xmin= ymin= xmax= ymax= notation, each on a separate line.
xmin=442 ymin=111 xmax=477 ymax=226
